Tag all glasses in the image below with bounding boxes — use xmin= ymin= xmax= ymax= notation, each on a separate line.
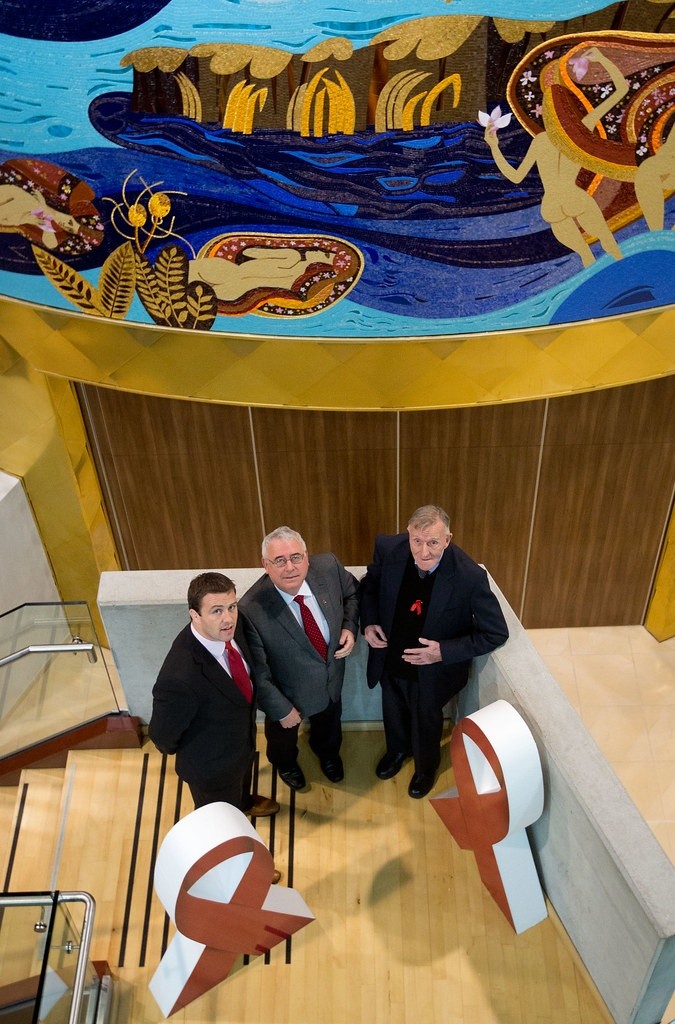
xmin=266 ymin=551 xmax=304 ymax=568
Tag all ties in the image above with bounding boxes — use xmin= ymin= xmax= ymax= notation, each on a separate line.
xmin=294 ymin=595 xmax=329 ymax=664
xmin=416 ymin=564 xmax=430 ymax=579
xmin=225 ymin=641 xmax=253 ymax=704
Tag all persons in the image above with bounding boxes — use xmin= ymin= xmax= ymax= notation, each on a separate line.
xmin=359 ymin=505 xmax=511 ymax=800
xmin=150 ymin=571 xmax=282 ymax=885
xmin=236 ymin=527 xmax=359 ymax=791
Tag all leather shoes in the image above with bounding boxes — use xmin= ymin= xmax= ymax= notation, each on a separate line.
xmin=409 ymin=751 xmax=440 ymax=797
xmin=278 ymin=759 xmax=306 ymax=790
xmin=272 ymin=870 xmax=281 ymax=884
xmin=319 ymin=752 xmax=344 ymax=783
xmin=241 ymin=794 xmax=280 ymax=817
xmin=376 ymin=747 xmax=416 ymax=780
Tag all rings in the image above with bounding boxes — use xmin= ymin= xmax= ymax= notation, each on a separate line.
xmin=350 ymin=651 xmax=351 ymax=653
